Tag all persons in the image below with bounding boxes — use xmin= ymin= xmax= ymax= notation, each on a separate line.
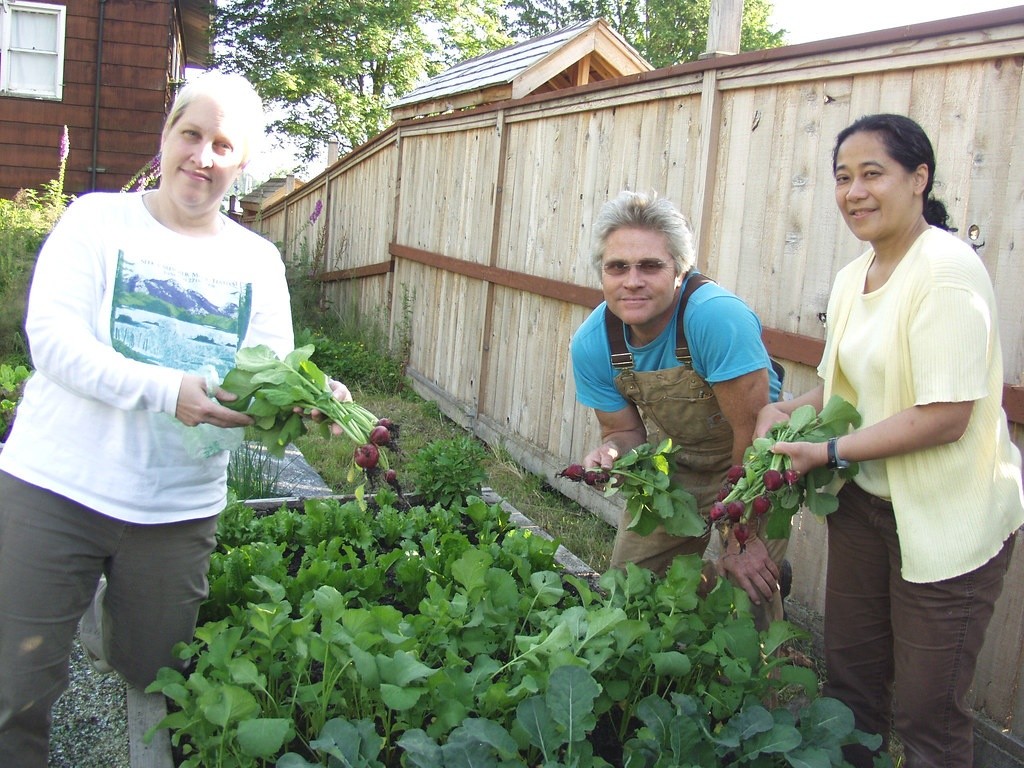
xmin=571 ymin=191 xmax=795 ymax=630
xmin=0 ymin=72 xmax=351 ymax=768
xmin=749 ymin=113 xmax=1024 ymax=768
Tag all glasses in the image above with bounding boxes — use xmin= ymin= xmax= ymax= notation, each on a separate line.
xmin=599 ymin=258 xmax=677 ymax=276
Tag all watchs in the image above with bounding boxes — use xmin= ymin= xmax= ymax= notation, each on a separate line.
xmin=825 ymin=437 xmax=849 ymax=472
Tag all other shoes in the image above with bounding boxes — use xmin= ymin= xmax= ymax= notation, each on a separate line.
xmin=780 ymin=559 xmax=792 ymax=621
xmin=75 ymin=575 xmax=108 ymax=674
